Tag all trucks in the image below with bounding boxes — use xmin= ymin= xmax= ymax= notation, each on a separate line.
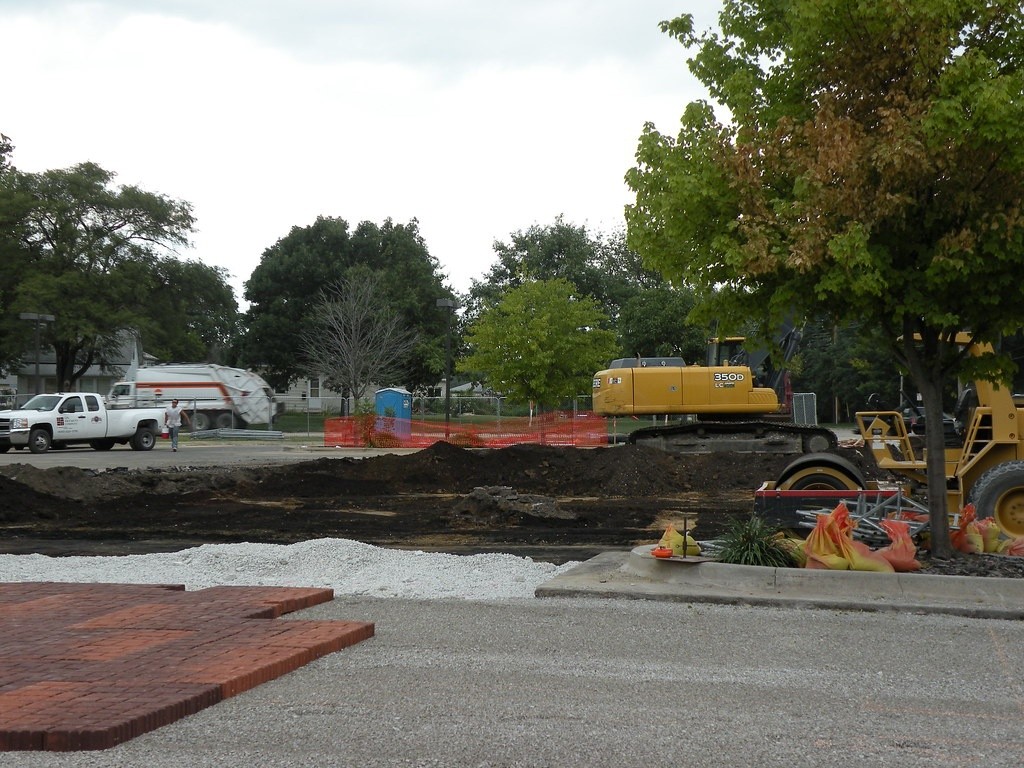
xmin=108 ymin=364 xmax=277 ymax=433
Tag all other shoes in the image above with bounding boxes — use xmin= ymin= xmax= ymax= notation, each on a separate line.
xmin=172 ymin=447 xmax=177 ymax=452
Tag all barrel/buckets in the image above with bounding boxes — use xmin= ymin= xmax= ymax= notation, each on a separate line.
xmin=161 ymin=428 xmax=169 ymax=439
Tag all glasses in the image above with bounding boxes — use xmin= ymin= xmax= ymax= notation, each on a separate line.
xmin=172 ymin=401 xmax=177 ymax=404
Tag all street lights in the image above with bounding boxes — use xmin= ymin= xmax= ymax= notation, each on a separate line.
xmin=436 ymin=297 xmax=462 ymax=442
xmin=19 ymin=311 xmax=56 ymax=396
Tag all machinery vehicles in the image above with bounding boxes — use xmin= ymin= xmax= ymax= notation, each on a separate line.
xmin=591 ymin=309 xmax=843 ymax=461
xmin=749 ymin=328 xmax=1024 ymax=546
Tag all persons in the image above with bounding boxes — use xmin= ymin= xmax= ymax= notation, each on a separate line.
xmin=165 ymin=399 xmax=191 ymax=452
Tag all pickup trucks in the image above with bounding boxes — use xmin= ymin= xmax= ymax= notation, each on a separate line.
xmin=0 ymin=393 xmax=168 ymax=454
